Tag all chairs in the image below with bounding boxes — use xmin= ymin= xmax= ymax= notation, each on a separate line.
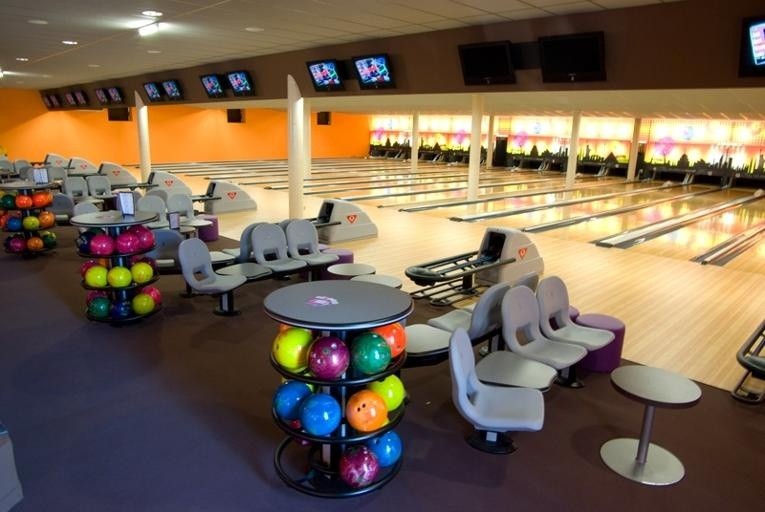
xmin=73 ymin=203 xmax=99 ymax=235
xmin=59 ymin=176 xmax=105 ymax=207
xmin=447 ymin=328 xmax=544 ymax=456
xmin=168 ymin=194 xmax=194 ymax=218
xmin=138 ymin=197 xmax=170 ymax=231
xmin=222 ymin=223 xmax=261 ymax=263
xmin=426 ymin=282 xmax=511 ymax=356
xmin=143 ymin=190 xmax=168 ymax=201
xmin=86 ymin=174 xmax=118 ymax=209
xmin=251 ymin=222 xmax=307 ymax=277
xmin=0 ymin=158 xmax=66 ymax=180
xmin=286 ymin=220 xmax=340 ymax=281
xmin=178 ymin=239 xmax=247 ymax=316
xmin=476 ymin=270 xmax=540 ymax=357
xmin=152 ymin=230 xmax=198 ymax=298
xmin=536 ymin=275 xmax=615 ymax=389
xmin=49 ymin=196 xmax=76 ymax=226
xmin=279 ymin=220 xmax=298 ymax=232
xmin=502 ymin=285 xmax=588 ymax=370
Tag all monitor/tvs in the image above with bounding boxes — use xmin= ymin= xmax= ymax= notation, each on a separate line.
xmin=225 ymin=70 xmax=257 ymax=97
xmin=161 ymin=79 xmax=182 ymax=100
xmin=93 ymin=88 xmax=109 ymax=105
xmin=537 ymin=32 xmax=608 ymax=84
xmin=143 ymin=81 xmax=164 ymax=103
xmin=306 ymin=58 xmax=346 ymax=93
xmin=739 ymin=17 xmax=765 ymax=75
xmin=74 ymin=89 xmax=86 ymax=105
xmin=458 ymin=40 xmax=518 ymax=86
xmin=44 ymin=95 xmax=54 ymax=108
xmin=64 ymin=91 xmax=76 ymax=106
xmin=351 ymin=52 xmax=397 ymax=90
xmin=199 ymin=73 xmax=227 ymax=99
xmin=108 ymin=87 xmax=124 ymax=104
xmin=49 ymin=94 xmax=61 ymax=107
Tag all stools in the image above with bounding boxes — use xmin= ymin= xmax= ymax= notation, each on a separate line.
xmin=216 ymin=262 xmax=273 ymax=283
xmin=568 ymin=306 xmax=579 ymax=321
xmin=197 ymin=215 xmax=219 ymax=242
xmin=576 ymin=312 xmax=625 ymax=373
xmin=318 ymin=243 xmax=328 ymax=252
xmin=473 ymin=350 xmax=559 ymax=397
xmin=209 ymin=250 xmax=235 ymax=269
xmin=403 ymin=325 xmax=456 ymax=366
xmin=322 ymin=248 xmax=355 ymax=265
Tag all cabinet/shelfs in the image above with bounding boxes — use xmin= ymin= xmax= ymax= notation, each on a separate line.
xmin=0 ymin=180 xmax=58 ymax=257
xmin=260 ymin=279 xmax=415 ymax=499
xmin=69 ymin=211 xmax=163 ymax=329
